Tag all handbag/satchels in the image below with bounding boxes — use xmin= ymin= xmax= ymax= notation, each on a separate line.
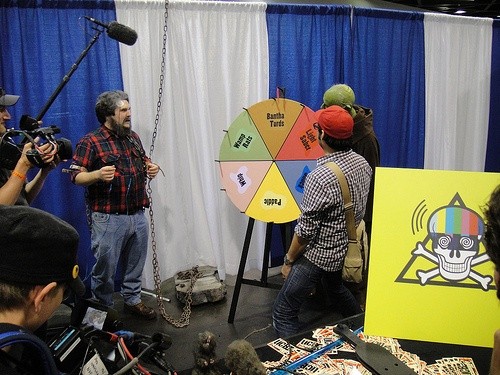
xmin=340 ymin=220 xmax=371 ymax=284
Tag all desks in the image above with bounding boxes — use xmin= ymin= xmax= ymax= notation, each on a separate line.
xmin=175 ymin=311 xmax=493 ymax=375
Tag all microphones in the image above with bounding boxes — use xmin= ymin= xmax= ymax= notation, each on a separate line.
xmin=84 ymin=15 xmax=138 ymax=47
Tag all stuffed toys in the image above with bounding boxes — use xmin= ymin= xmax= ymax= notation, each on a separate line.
xmin=225 ymin=339 xmax=267 ymax=375
xmin=193 ymin=331 xmax=220 ymax=375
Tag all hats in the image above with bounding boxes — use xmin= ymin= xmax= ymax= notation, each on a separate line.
xmin=323 ymin=84 xmax=356 ymax=110
xmin=0 ymin=204 xmax=89 ymax=295
xmin=318 ymin=105 xmax=354 ymax=140
xmin=0 ymin=87 xmax=20 ymax=107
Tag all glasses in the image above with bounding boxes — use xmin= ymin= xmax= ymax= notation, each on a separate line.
xmin=312 ymin=122 xmax=322 ymax=130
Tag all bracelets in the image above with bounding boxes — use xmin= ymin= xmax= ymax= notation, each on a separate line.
xmin=11 ymin=170 xmax=25 ymax=181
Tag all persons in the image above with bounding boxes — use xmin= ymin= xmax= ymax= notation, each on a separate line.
xmin=273 ymin=104 xmax=371 ymax=340
xmin=68 ymin=90 xmax=159 ymax=320
xmin=481 ymin=185 xmax=500 ymax=375
xmin=0 ymin=86 xmax=86 ymax=375
xmin=320 ymin=83 xmax=379 ymax=296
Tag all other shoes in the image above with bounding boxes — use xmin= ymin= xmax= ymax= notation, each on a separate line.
xmin=123 ymin=300 xmax=159 ymax=319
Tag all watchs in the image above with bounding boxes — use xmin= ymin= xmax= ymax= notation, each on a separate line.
xmin=284 ymin=253 xmax=294 ymax=265
